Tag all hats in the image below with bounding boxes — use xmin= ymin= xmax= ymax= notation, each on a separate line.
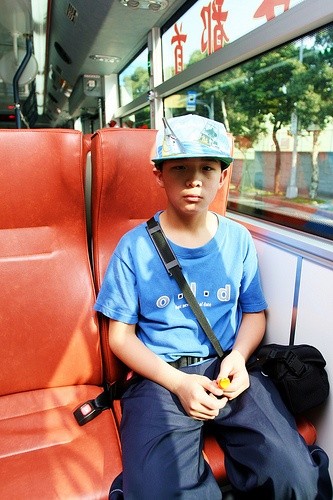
xmin=152 ymin=115 xmax=233 ymax=164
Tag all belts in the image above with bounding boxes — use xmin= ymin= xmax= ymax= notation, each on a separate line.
xmin=168 ymin=350 xmax=208 ymax=368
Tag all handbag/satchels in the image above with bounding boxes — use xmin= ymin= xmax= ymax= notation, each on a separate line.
xmin=249 ymin=344 xmax=329 ymax=414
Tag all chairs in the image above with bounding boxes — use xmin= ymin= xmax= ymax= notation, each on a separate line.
xmin=91 ymin=128 xmax=319 ymax=484
xmin=0 ymin=128 xmax=129 ymax=500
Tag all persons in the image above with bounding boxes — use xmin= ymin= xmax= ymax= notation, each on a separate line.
xmin=93 ymin=114 xmax=333 ymax=500
